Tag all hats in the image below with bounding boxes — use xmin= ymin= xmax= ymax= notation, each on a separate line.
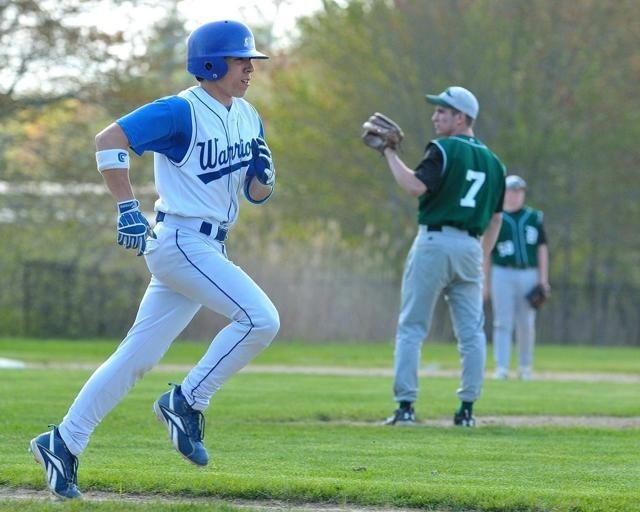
xmin=505 ymin=175 xmax=526 ymax=189
xmin=425 ymin=86 xmax=480 ymax=120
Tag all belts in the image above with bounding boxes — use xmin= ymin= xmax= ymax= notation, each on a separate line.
xmin=157 ymin=211 xmax=227 ymax=241
xmin=427 ymin=224 xmax=477 ymax=238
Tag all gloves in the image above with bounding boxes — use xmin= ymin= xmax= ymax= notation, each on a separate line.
xmin=251 ymin=137 xmax=276 ymax=186
xmin=117 ymin=199 xmax=157 ymax=257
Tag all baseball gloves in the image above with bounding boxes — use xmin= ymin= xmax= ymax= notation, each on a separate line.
xmin=525 ymin=284 xmax=546 ymax=310
xmin=361 ymin=112 xmax=404 ymax=156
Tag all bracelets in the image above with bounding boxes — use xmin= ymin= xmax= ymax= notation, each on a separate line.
xmin=94 ymin=149 xmax=131 ymax=173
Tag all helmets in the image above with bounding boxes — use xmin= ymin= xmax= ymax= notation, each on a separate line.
xmin=187 ymin=21 xmax=269 ymax=81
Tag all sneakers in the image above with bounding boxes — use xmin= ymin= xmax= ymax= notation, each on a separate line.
xmin=153 ymin=382 xmax=208 ymax=466
xmin=495 ymin=371 xmax=508 ymax=379
xmin=30 ymin=423 xmax=83 ymax=500
xmin=519 ymin=369 xmax=532 ymax=380
xmin=383 ymin=407 xmax=415 ymax=424
xmin=454 ymin=413 xmax=474 ymax=427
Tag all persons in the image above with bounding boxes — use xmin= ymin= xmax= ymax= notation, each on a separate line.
xmin=481 ymin=173 xmax=551 ymax=382
xmin=359 ymin=85 xmax=507 ymax=428
xmin=27 ymin=20 xmax=281 ymax=501
